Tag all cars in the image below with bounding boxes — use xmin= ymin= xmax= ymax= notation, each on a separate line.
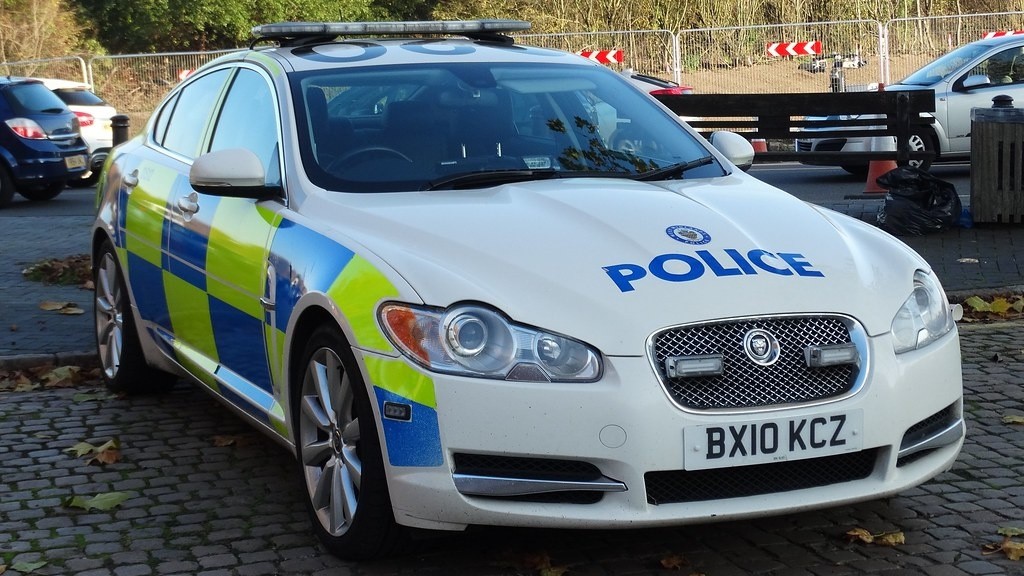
xmin=798 ymin=52 xmax=867 ymax=74
xmin=794 ymin=31 xmax=1024 ymax=187
xmin=89 ymin=19 xmax=968 ymax=566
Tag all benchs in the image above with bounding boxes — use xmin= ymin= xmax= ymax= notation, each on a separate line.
xmin=649 ymin=89 xmax=937 ymax=169
xmin=329 ymin=101 xmax=450 ymax=166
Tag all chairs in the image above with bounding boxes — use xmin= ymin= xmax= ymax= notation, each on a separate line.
xmin=1011 ymin=54 xmax=1024 ymax=83
xmin=450 ymin=104 xmax=520 ymax=174
xmin=306 ymin=86 xmax=354 ymax=171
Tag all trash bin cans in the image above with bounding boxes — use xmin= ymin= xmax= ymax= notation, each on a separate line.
xmin=968 ymin=107 xmax=1024 ymax=224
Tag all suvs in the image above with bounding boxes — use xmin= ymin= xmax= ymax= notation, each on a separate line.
xmin=0 ymin=76 xmax=94 ymax=209
xmin=0 ymin=76 xmax=118 ymax=188
